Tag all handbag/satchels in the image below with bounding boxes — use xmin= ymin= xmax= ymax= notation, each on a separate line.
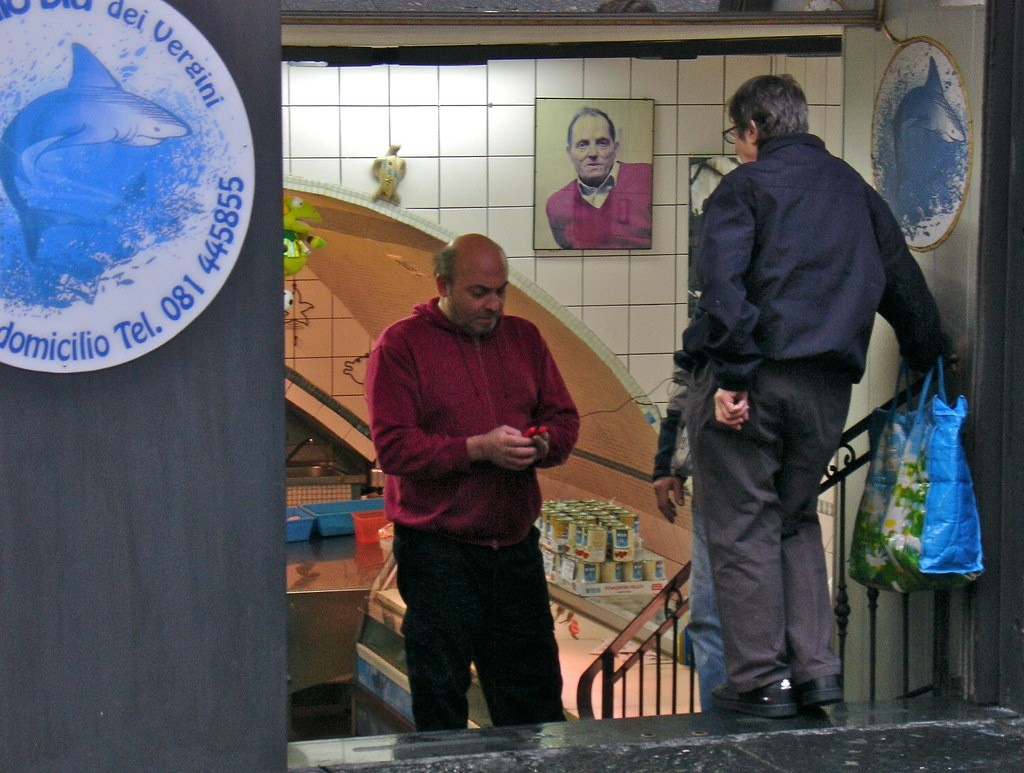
xmin=848 ymin=357 xmax=984 ymax=594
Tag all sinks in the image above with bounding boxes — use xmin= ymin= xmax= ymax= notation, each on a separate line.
xmin=286 ymin=466 xmax=343 ymax=482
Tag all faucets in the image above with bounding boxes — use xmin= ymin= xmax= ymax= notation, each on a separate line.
xmin=287 ymin=438 xmax=314 ymax=460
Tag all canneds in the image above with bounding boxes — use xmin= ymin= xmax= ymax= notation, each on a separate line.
xmin=538 ymin=498 xmax=665 ymax=582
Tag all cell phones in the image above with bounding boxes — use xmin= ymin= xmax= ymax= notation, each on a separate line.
xmin=522 ymin=425 xmax=547 ymax=437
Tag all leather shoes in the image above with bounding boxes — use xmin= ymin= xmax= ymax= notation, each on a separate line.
xmin=712 ymin=676 xmax=796 ymax=715
xmin=793 ymin=674 xmax=844 ymax=705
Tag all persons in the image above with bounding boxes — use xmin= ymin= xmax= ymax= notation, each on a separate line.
xmin=368 ymin=233 xmax=580 ymax=732
xmin=549 ymin=109 xmax=652 ymax=249
xmin=652 ymin=353 xmax=728 ymax=713
xmin=682 ymin=75 xmax=943 ymax=712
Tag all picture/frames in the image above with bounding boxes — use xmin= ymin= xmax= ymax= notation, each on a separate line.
xmin=532 ymin=97 xmax=655 ymax=251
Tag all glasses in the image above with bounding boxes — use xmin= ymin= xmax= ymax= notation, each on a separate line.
xmin=722 ymin=121 xmax=747 ymax=144
